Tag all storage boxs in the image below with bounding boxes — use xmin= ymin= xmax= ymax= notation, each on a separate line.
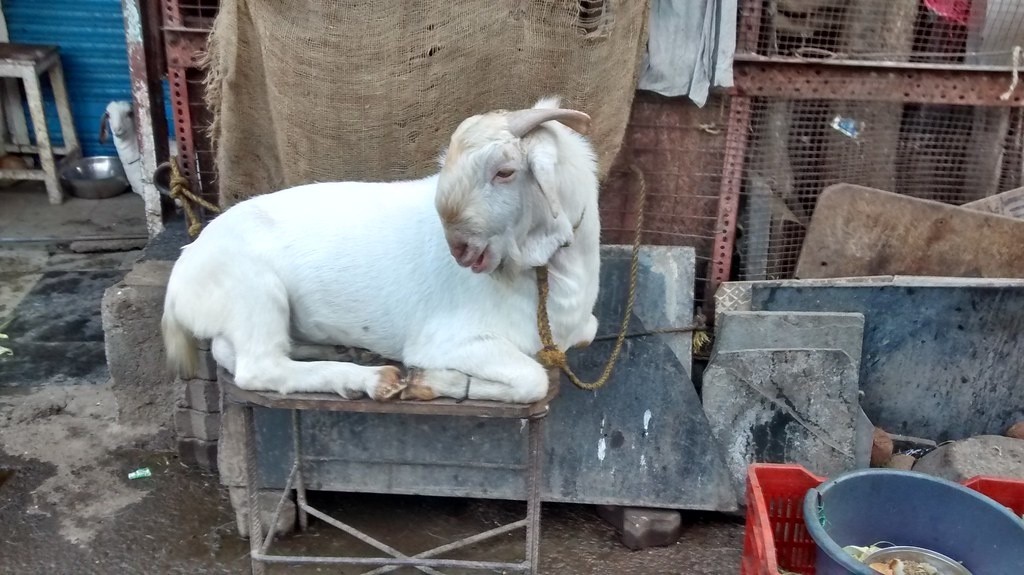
xmin=742 ymin=462 xmax=1024 ymax=575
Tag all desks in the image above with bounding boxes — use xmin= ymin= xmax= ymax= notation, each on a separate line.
xmin=242 ymin=353 xmax=570 ymax=573
xmin=1 ymin=42 xmax=85 ymax=208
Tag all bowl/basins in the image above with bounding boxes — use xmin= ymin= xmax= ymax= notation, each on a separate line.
xmin=59 ymin=153 xmax=131 ymax=199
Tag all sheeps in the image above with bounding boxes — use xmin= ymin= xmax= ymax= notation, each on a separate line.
xmin=161 ymin=93 xmax=601 ymax=404
xmin=98 ymin=101 xmax=144 ymax=198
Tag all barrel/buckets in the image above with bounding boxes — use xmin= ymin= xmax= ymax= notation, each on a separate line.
xmin=806 ymin=465 xmax=1024 ymax=575
